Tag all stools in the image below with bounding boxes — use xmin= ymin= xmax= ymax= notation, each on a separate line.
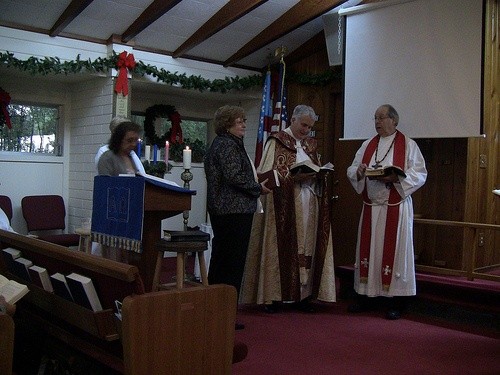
xmin=77 ymin=228 xmax=115 ymax=261
xmin=150 ymin=238 xmax=208 ymax=294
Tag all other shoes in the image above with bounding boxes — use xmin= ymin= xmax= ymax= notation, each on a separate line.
xmin=386 ymin=302 xmax=402 ymax=319
xmin=348 ymin=298 xmax=379 ymax=314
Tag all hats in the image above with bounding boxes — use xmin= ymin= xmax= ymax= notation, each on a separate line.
xmin=110 ymin=116 xmax=131 ymax=131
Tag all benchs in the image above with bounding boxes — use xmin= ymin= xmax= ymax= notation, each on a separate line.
xmin=0 ymin=228 xmax=238 ymax=375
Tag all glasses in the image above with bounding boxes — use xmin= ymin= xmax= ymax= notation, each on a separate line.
xmin=236 ymin=119 xmax=247 ymax=125
xmin=374 ymin=116 xmax=387 ymax=122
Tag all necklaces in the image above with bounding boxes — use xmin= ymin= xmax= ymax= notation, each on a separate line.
xmin=373 ymin=137 xmax=395 ymax=169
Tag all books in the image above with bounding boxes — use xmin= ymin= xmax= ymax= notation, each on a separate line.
xmin=289 ymin=160 xmax=334 ymax=175
xmin=0 ymin=275 xmax=30 ymax=305
xmin=366 ymin=166 xmax=406 ymax=180
xmin=258 ymin=169 xmax=280 ymax=190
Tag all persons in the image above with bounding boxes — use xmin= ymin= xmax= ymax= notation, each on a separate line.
xmin=0 ymin=209 xmax=38 ymax=238
xmin=0 ymin=304 xmax=43 ymax=375
xmin=205 ymin=106 xmax=273 ymax=330
xmin=238 ymin=106 xmax=336 ymax=313
xmin=347 ymin=104 xmax=427 ymax=320
xmin=92 ymin=122 xmax=142 ymax=256
xmin=95 ymin=115 xmax=145 ymax=174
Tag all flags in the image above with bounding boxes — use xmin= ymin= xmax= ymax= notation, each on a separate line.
xmin=254 ymin=72 xmax=273 ymax=168
xmin=271 ymin=63 xmax=287 ymax=135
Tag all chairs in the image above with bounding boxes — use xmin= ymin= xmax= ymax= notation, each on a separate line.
xmin=22 ymin=194 xmax=83 ymax=248
xmin=0 ymin=193 xmax=13 ymax=225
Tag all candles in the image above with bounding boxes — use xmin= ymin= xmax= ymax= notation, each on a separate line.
xmin=154 ymin=144 xmax=158 ymax=162
xmin=137 ymin=138 xmax=141 ymax=158
xmin=165 ymin=140 xmax=169 ymax=165
xmin=183 ymin=145 xmax=192 ymax=169
xmin=145 ymin=145 xmax=150 ymax=164
